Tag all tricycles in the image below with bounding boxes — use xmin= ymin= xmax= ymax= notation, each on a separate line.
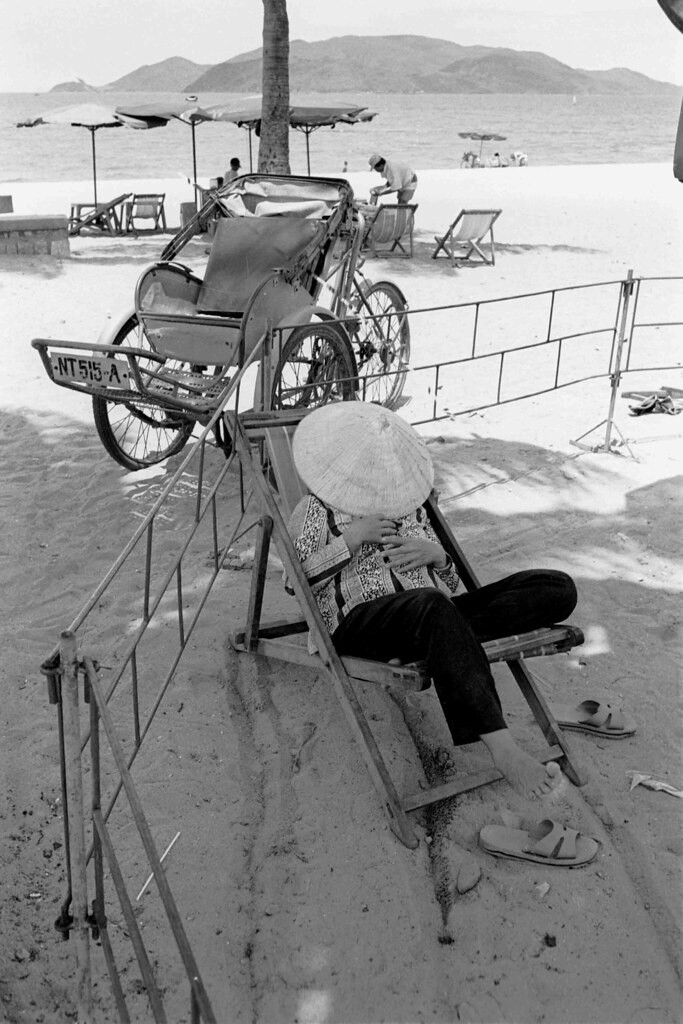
xmin=31 ymin=168 xmax=413 ymax=477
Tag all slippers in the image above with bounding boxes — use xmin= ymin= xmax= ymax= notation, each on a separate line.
xmin=547 ymin=699 xmax=637 ymax=739
xmin=479 ymin=819 xmax=598 ymax=865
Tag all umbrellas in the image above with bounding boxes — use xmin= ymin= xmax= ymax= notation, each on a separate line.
xmin=458 ymin=131 xmax=507 ymax=160
xmin=113 ymin=104 xmax=208 ymax=204
xmin=16 ymin=105 xmax=166 ymax=212
xmin=181 ymin=93 xmax=379 ymax=176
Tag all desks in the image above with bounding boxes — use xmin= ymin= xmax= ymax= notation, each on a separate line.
xmin=71 ymin=204 xmax=118 ymax=235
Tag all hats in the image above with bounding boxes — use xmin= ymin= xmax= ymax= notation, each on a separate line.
xmin=369 ymin=155 xmax=381 ymax=171
xmin=292 ymin=401 xmax=434 ymax=516
xmin=230 ymin=158 xmax=241 ymax=168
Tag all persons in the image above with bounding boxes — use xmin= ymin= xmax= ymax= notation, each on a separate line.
xmin=491 ymin=153 xmax=501 ymax=166
xmin=217 ymin=177 xmax=224 ymax=189
xmin=224 ymin=158 xmax=242 ymax=184
xmin=284 ymin=401 xmax=578 ymax=801
xmin=368 ymin=155 xmax=418 ymax=204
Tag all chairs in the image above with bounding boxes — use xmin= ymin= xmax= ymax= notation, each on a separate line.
xmin=433 ymin=208 xmax=502 ymax=268
xmin=138 ymin=215 xmax=325 ymax=364
xmin=229 ymin=400 xmax=588 ymax=849
xmin=362 ymin=202 xmax=419 ymax=257
xmin=68 ymin=193 xmax=166 ymax=236
